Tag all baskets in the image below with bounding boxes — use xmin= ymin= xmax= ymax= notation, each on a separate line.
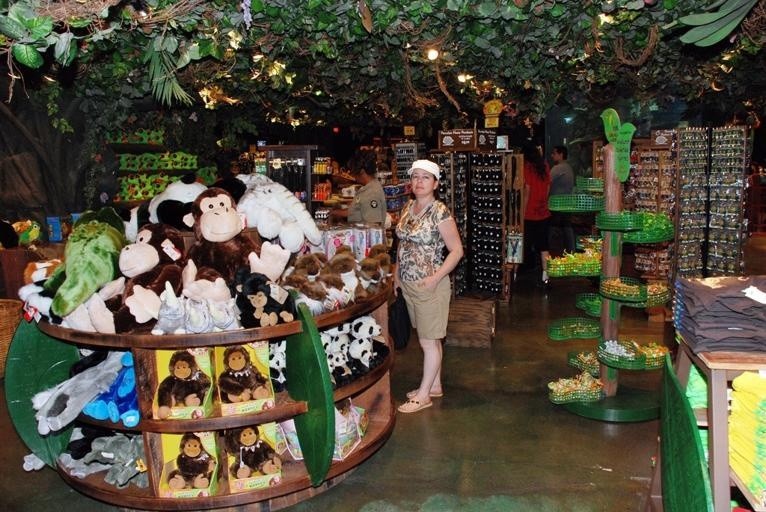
xmin=0 ymin=299 xmax=24 ymax=380
xmin=548 ymin=175 xmax=674 ymax=405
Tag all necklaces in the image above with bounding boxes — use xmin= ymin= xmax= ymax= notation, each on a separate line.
xmin=409 ymin=196 xmax=437 ymax=219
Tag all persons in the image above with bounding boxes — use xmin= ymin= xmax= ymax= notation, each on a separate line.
xmin=517 ymin=142 xmax=554 ymax=288
xmin=398 ymin=159 xmax=466 ymax=416
xmin=745 ymin=164 xmax=756 ymax=190
xmin=546 ymin=143 xmax=575 ymax=261
xmin=345 ymin=148 xmax=389 ymax=228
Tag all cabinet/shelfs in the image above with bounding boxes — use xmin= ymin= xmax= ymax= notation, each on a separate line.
xmin=108 ymin=166 xmax=195 ymax=207
xmin=427 ymin=148 xmax=526 ymax=305
xmin=589 ymin=125 xmax=754 ymax=281
xmin=30 ymin=292 xmax=395 ymax=510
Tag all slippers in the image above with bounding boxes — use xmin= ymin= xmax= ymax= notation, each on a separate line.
xmin=407 ymin=388 xmax=443 ymax=398
xmin=397 ymin=396 xmax=433 ymax=414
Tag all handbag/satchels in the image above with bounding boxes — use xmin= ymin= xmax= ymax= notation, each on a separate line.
xmin=388 ymin=288 xmax=410 ymax=351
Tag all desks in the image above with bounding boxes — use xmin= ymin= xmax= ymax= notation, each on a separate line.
xmin=644 ymin=329 xmax=766 ymax=512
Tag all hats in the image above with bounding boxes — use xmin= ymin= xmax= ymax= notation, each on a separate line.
xmin=408 ymin=160 xmax=439 ymax=180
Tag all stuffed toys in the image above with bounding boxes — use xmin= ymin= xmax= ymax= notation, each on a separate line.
xmin=2 ymin=158 xmax=395 ymax=500
xmin=3 ymin=158 xmax=395 ymax=501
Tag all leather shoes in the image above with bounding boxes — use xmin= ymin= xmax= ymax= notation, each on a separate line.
xmin=536 ymin=278 xmax=551 ymax=287
